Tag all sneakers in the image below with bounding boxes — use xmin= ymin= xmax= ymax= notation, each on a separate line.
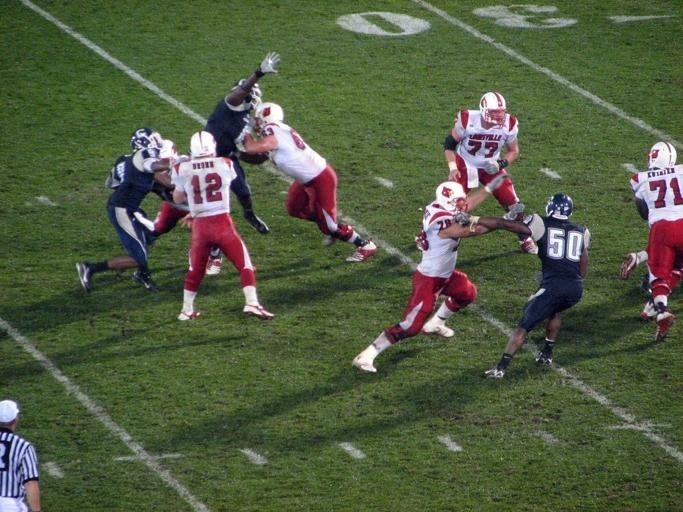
xmin=483 ymin=366 xmax=505 ymax=378
xmin=178 ymin=310 xmax=202 ymax=322
xmin=76 ymin=261 xmax=94 ymax=293
xmin=322 ymin=235 xmax=339 ymax=246
xmin=352 ymin=356 xmax=377 ymax=373
xmin=621 ymin=253 xmax=637 ymax=278
xmin=422 ymin=324 xmax=455 ymax=337
xmin=209 ymin=245 xmax=223 ymax=260
xmin=242 ymin=301 xmax=274 ymax=320
xmin=521 ymin=237 xmax=539 ymax=254
xmin=133 ymin=271 xmax=157 ymax=290
xmin=653 ymin=312 xmax=674 ymax=343
xmin=640 ymin=300 xmax=659 ymax=319
xmin=535 ymin=353 xmax=552 ymax=365
xmin=206 ymin=258 xmax=223 ymax=275
xmin=344 ymin=241 xmax=379 ymax=262
xmin=415 ymin=231 xmax=429 ymax=250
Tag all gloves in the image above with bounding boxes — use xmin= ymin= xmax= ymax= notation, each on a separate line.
xmin=482 ymin=159 xmax=500 ymax=175
xmin=454 ymin=211 xmax=473 ymax=224
xmin=170 ymin=154 xmax=188 ymax=168
xmin=260 ymin=52 xmax=280 ymax=73
xmin=503 ymin=203 xmax=526 ymax=221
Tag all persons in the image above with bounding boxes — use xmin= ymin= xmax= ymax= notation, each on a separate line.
xmin=631 ymin=142 xmax=683 ymax=342
xmin=415 ymin=91 xmax=540 ymax=256
xmin=0 ymin=399 xmax=41 ymax=512
xmin=455 ymin=193 xmax=590 ymax=377
xmin=242 ymin=102 xmax=377 ymax=262
xmin=622 ymin=244 xmax=683 ymax=283
xmin=75 ymin=127 xmax=188 ymax=293
xmin=152 ymin=137 xmax=223 ymax=276
xmin=350 ymin=182 xmax=525 ymax=374
xmin=174 ymin=132 xmax=275 ymax=321
xmin=205 ymin=49 xmax=280 ymax=237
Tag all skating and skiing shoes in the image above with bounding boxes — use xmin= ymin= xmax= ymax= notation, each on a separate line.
xmin=249 ymin=217 xmax=268 ymax=233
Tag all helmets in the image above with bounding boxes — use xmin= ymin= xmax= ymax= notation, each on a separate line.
xmin=232 ymin=78 xmax=262 ymax=111
xmin=131 ymin=127 xmax=164 ymax=152
xmin=254 ymin=102 xmax=287 ymax=128
xmin=479 ymin=92 xmax=506 ymax=125
xmin=436 ymin=182 xmax=467 ymax=213
xmin=546 ymin=191 xmax=574 ymax=220
xmin=188 ymin=129 xmax=217 ymax=160
xmin=648 ymin=141 xmax=677 ymax=170
xmin=159 ymin=140 xmax=180 ymax=161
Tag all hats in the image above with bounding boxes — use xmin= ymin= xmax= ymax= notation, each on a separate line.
xmin=0 ymin=400 xmax=19 ymax=423
